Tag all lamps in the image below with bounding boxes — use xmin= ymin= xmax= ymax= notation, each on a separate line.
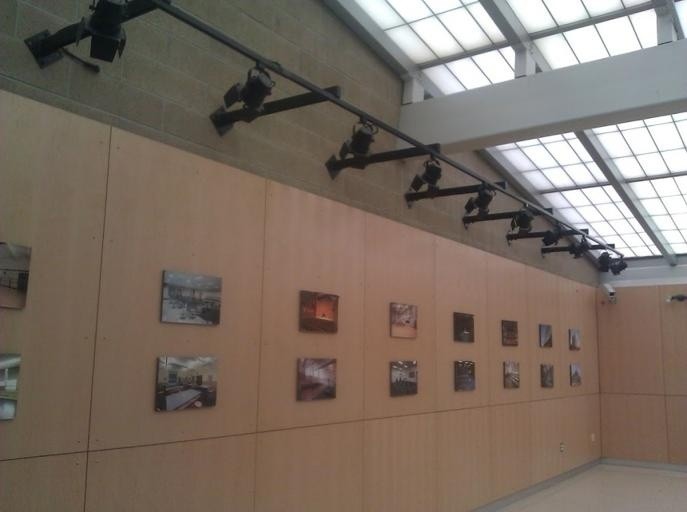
xmin=12 ymin=2 xmax=629 ymax=274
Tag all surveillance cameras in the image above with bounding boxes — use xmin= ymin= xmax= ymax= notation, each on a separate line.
xmin=602 ymin=283 xmax=617 ymax=297
